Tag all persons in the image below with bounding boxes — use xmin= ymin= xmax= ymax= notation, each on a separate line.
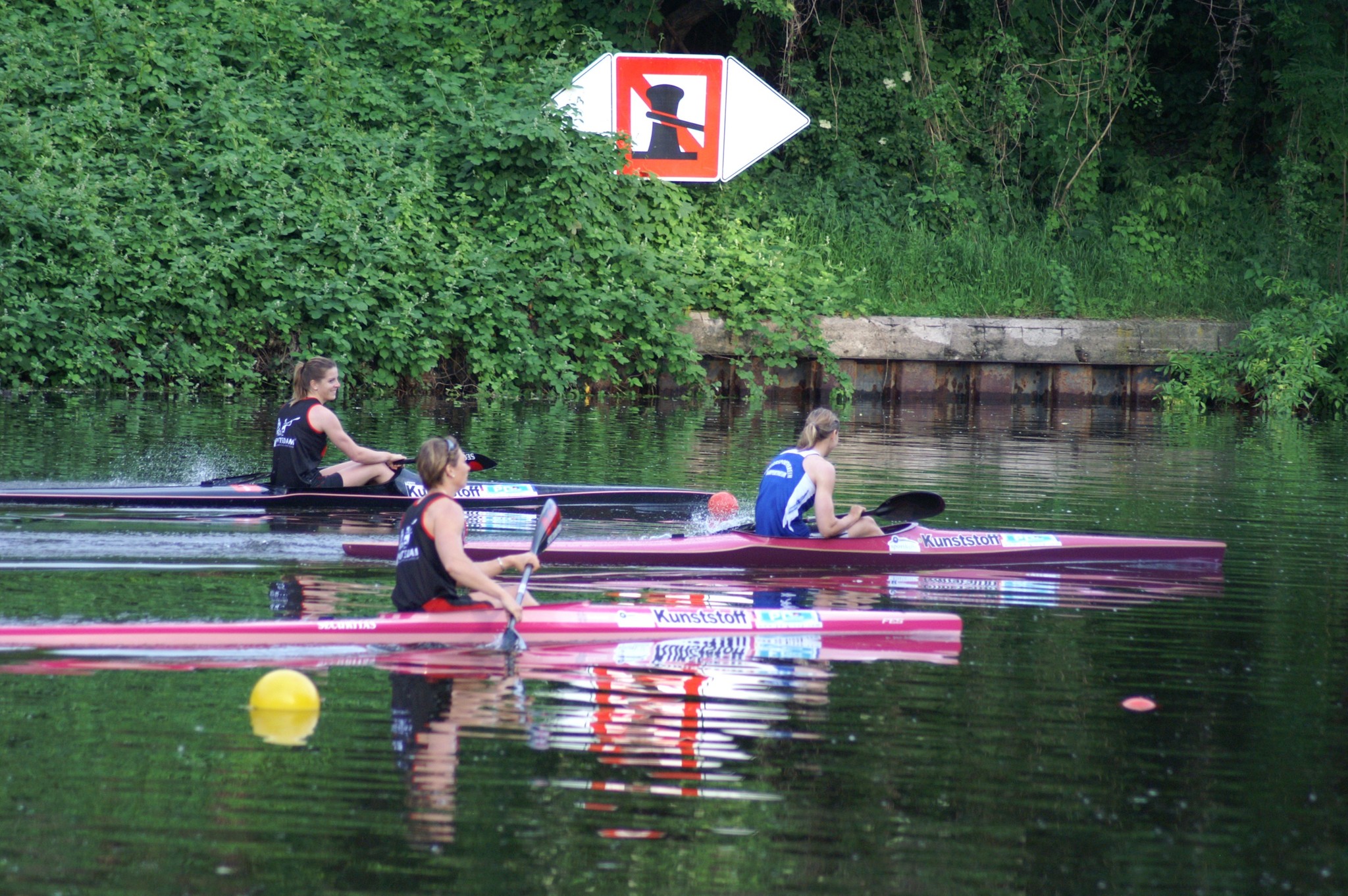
xmin=270 ymin=357 xmax=407 ymax=488
xmin=755 ymin=408 xmax=885 ymax=541
xmin=391 ymin=436 xmax=541 ymax=622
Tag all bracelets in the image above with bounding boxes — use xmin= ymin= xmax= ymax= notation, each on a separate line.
xmin=497 ymin=556 xmax=505 ymax=571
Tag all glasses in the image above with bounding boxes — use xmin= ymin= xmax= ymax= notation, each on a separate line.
xmin=434 ymin=435 xmax=457 ymax=466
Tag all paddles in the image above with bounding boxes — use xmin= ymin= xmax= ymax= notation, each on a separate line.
xmin=200 ymin=452 xmax=499 ymax=487
xmin=711 ymin=492 xmax=947 ymax=532
xmin=491 ymin=497 xmax=566 ymax=648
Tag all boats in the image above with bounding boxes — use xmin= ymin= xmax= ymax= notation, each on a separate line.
xmin=342 ymin=521 xmax=1227 ymax=565
xmin=1 ymin=605 xmax=964 ymax=648
xmin=1 ymin=466 xmax=717 ymax=505
